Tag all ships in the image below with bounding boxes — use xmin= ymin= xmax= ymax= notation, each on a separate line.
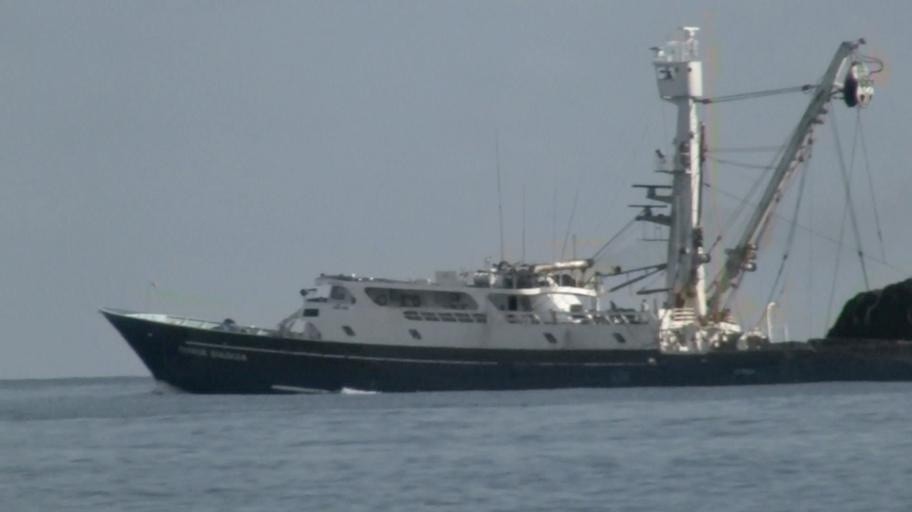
xmin=98 ymin=25 xmax=910 ymax=396
